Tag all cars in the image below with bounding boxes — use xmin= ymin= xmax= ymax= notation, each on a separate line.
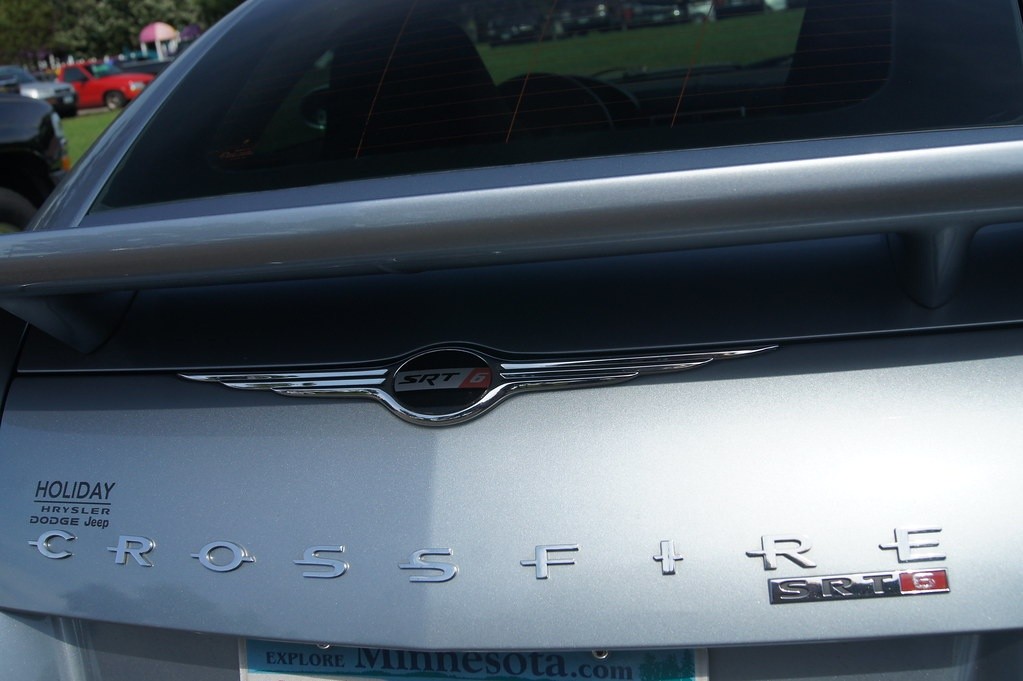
xmin=0 ymin=66 xmax=80 ymax=120
xmin=0 ymin=89 xmax=70 ymax=235
xmin=466 ymin=0 xmax=794 ymax=47
xmin=0 ymin=0 xmax=1023 ymax=681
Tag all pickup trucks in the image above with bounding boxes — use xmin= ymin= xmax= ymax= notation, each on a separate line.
xmin=58 ymin=61 xmax=154 ymax=115
